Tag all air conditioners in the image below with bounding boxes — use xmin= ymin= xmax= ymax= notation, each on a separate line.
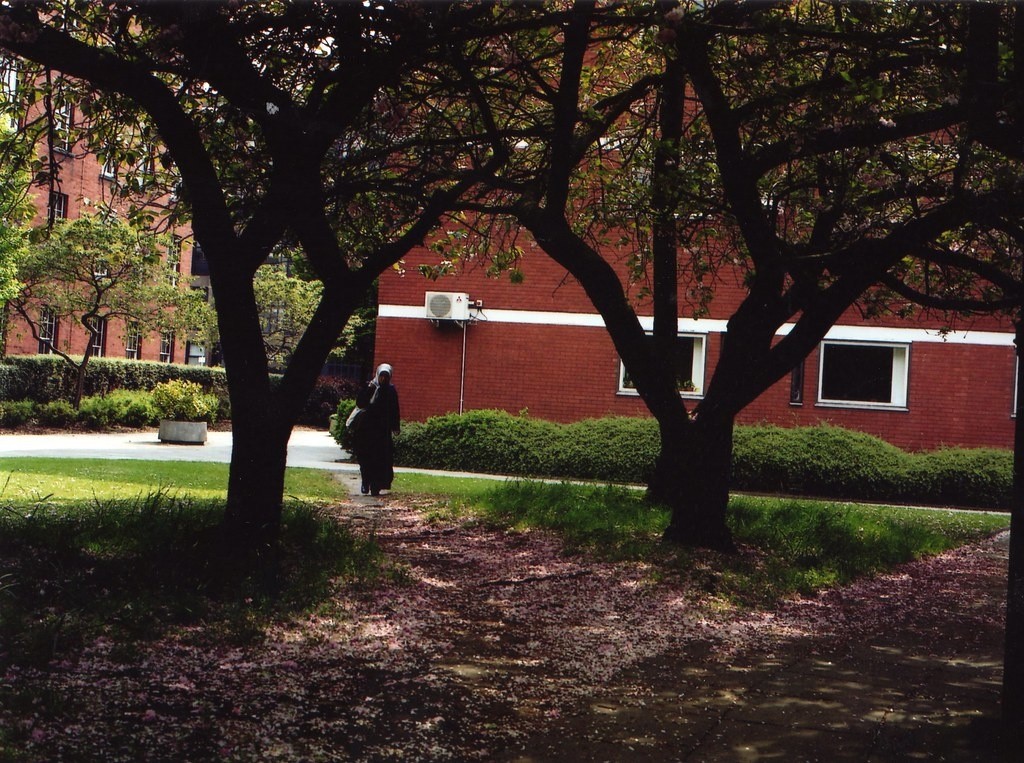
xmin=425 ymin=291 xmax=471 ymax=320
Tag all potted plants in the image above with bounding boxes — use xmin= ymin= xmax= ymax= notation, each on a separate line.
xmin=143 ymin=375 xmax=221 ymax=444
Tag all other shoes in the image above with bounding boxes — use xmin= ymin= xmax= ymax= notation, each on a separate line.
xmin=361 ymin=483 xmax=369 ymax=493
xmin=371 ymin=489 xmax=379 ymax=496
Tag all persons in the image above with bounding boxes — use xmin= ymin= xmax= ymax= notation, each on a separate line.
xmin=356 ymin=363 xmax=400 ymax=497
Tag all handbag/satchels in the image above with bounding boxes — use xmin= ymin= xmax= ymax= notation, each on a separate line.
xmin=345 ymin=406 xmax=366 ymax=432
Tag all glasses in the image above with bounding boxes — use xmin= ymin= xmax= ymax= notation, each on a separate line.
xmin=380 ymin=372 xmax=389 ymax=377
